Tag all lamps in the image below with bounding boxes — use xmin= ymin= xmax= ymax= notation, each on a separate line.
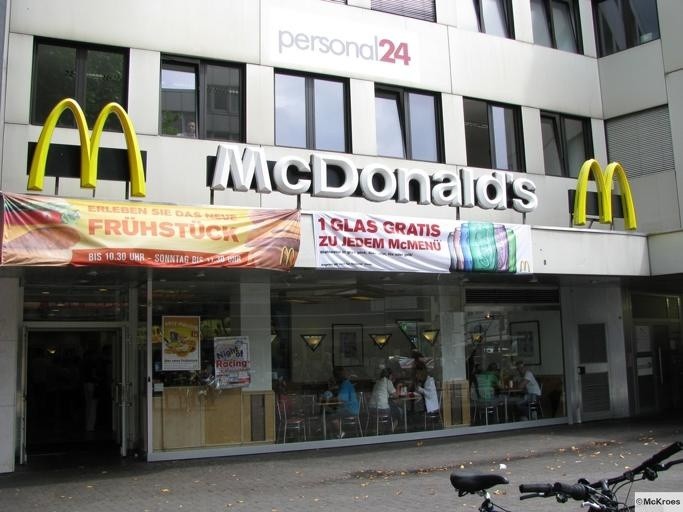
xmin=368 ymin=333 xmax=392 ymax=349
xmin=421 ymin=329 xmax=440 ymax=346
xmin=300 ymin=333 xmax=326 ymax=351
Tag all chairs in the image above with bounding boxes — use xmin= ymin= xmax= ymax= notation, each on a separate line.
xmin=470 ymin=381 xmax=543 ymax=426
xmin=275 ymin=386 xmax=444 ymax=443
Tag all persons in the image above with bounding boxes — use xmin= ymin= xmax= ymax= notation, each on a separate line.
xmin=176 ymin=120 xmax=196 ymax=138
xmin=406 ymin=362 xmax=438 ymax=431
xmin=328 ymin=366 xmax=359 ymax=438
xmin=468 ymin=355 xmax=481 ymax=400
xmin=513 ymin=360 xmax=542 ymax=422
xmin=477 ymin=362 xmax=510 ymax=422
xmin=81 ymin=350 xmax=98 ymax=432
xmin=369 ymin=366 xmax=401 ymax=435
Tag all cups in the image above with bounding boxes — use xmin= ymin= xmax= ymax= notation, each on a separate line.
xmin=509 ymin=380 xmax=513 ymax=388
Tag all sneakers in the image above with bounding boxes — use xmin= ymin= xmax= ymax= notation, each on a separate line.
xmin=337 ymin=431 xmax=346 ymax=439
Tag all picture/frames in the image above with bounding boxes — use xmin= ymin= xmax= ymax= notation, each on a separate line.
xmin=331 ymin=324 xmax=363 ymax=367
xmin=509 ymin=320 xmax=542 ymax=366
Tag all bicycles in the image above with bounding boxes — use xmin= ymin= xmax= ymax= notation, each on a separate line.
xmin=449 ymin=440 xmax=682 ymax=510
